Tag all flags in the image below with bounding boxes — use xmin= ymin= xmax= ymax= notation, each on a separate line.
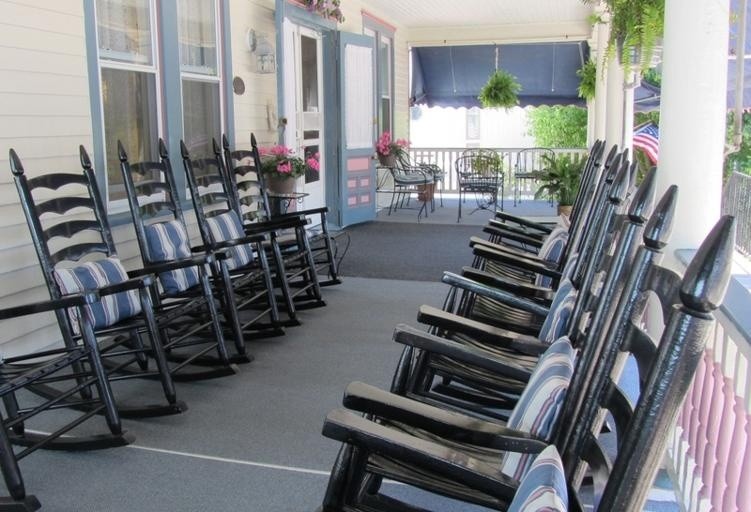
xmin=633 ymin=122 xmax=659 ymax=166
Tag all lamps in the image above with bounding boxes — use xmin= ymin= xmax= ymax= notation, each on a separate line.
xmin=246 ymin=26 xmax=276 ymax=74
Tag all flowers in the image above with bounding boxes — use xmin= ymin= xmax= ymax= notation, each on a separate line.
xmin=303 ymin=0 xmax=346 ymax=25
